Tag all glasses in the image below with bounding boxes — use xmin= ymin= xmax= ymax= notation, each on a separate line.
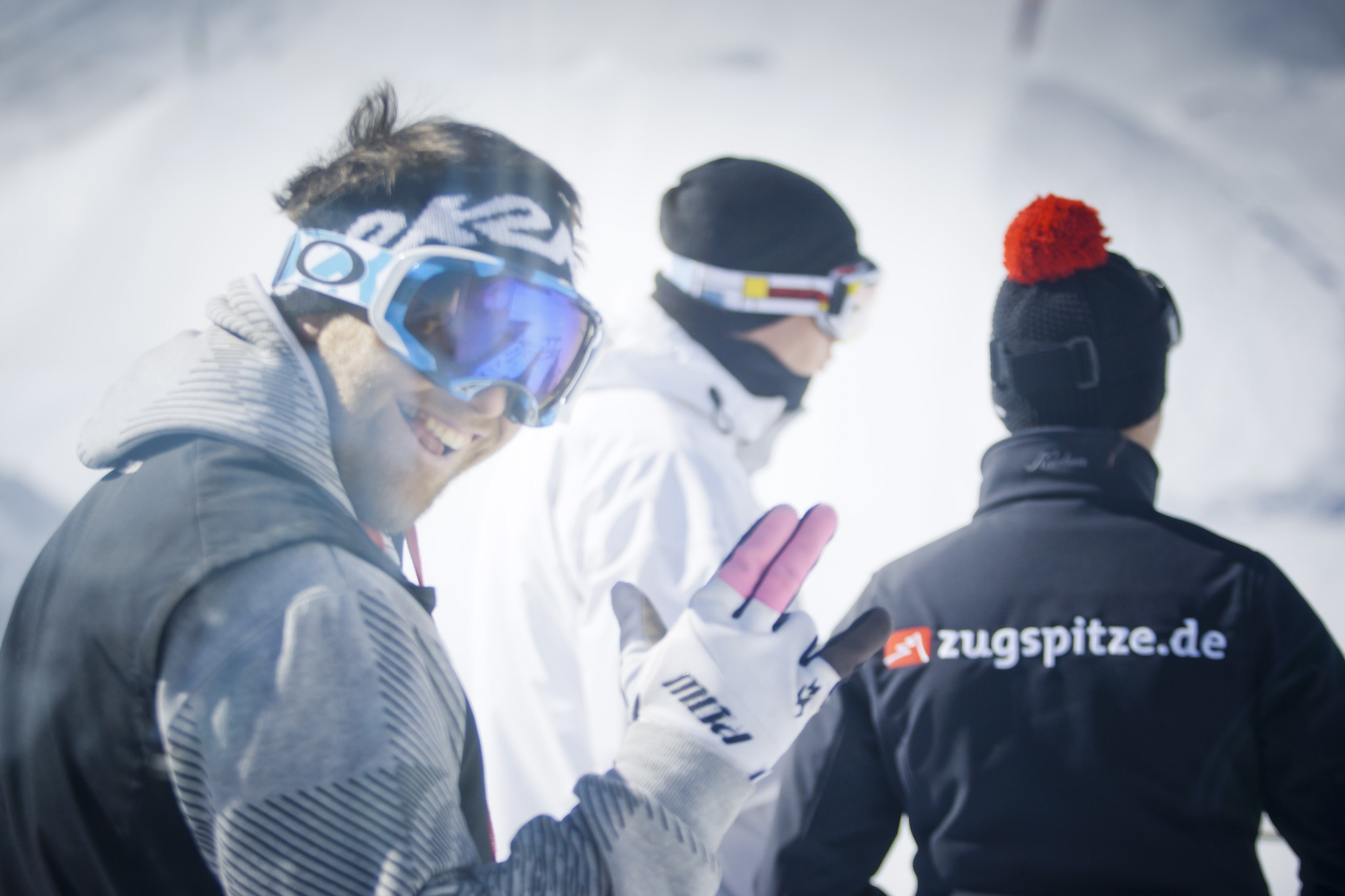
xmin=814 ymin=259 xmax=878 ymax=339
xmin=369 ymin=246 xmax=604 ymax=430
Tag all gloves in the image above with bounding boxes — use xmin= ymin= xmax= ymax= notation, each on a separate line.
xmin=609 ymin=502 xmax=891 ymax=787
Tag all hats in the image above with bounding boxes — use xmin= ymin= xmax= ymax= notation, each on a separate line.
xmin=987 ymin=191 xmax=1164 ymax=430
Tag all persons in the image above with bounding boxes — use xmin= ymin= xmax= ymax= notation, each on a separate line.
xmin=717 ymin=194 xmax=1344 ymax=895
xmin=482 ymin=157 xmax=886 ymax=896
xmin=0 ymin=77 xmax=891 ymax=896
